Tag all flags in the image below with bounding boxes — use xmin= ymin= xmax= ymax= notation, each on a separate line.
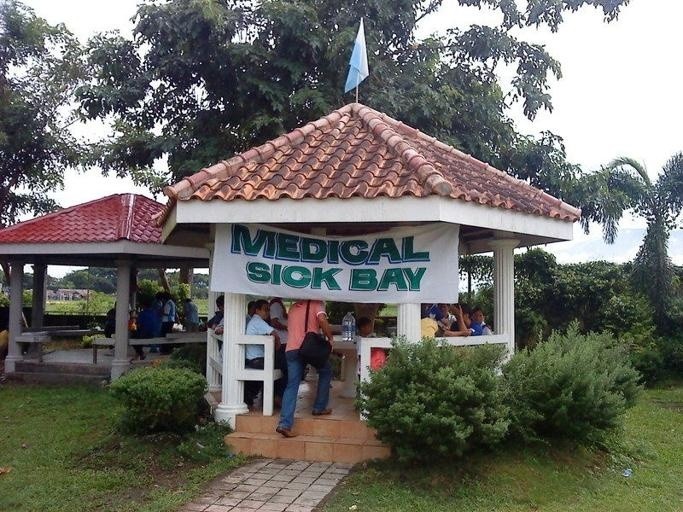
xmin=344 ymin=17 xmax=369 ymax=94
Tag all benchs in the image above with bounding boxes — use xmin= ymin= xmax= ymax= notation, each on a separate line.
xmin=208 ymin=327 xmax=508 ymax=420
xmin=20 ymin=320 xmax=210 ymax=366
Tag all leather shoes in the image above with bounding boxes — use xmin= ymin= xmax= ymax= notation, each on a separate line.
xmin=276 ymin=427 xmax=296 ymax=437
xmin=312 ymin=408 xmax=333 ymax=416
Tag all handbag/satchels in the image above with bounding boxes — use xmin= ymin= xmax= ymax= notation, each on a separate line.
xmin=299 ymin=331 xmax=333 ymax=370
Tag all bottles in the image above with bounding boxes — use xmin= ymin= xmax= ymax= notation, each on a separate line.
xmin=341 ymin=312 xmax=356 ymax=341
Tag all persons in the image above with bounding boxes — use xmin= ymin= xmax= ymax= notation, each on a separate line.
xmin=207 ymin=296 xmax=334 ymax=438
xmin=105 ymin=291 xmax=199 ymax=361
xmin=420 ymin=302 xmax=490 ymax=338
xmin=356 ymin=317 xmax=372 ymax=338
